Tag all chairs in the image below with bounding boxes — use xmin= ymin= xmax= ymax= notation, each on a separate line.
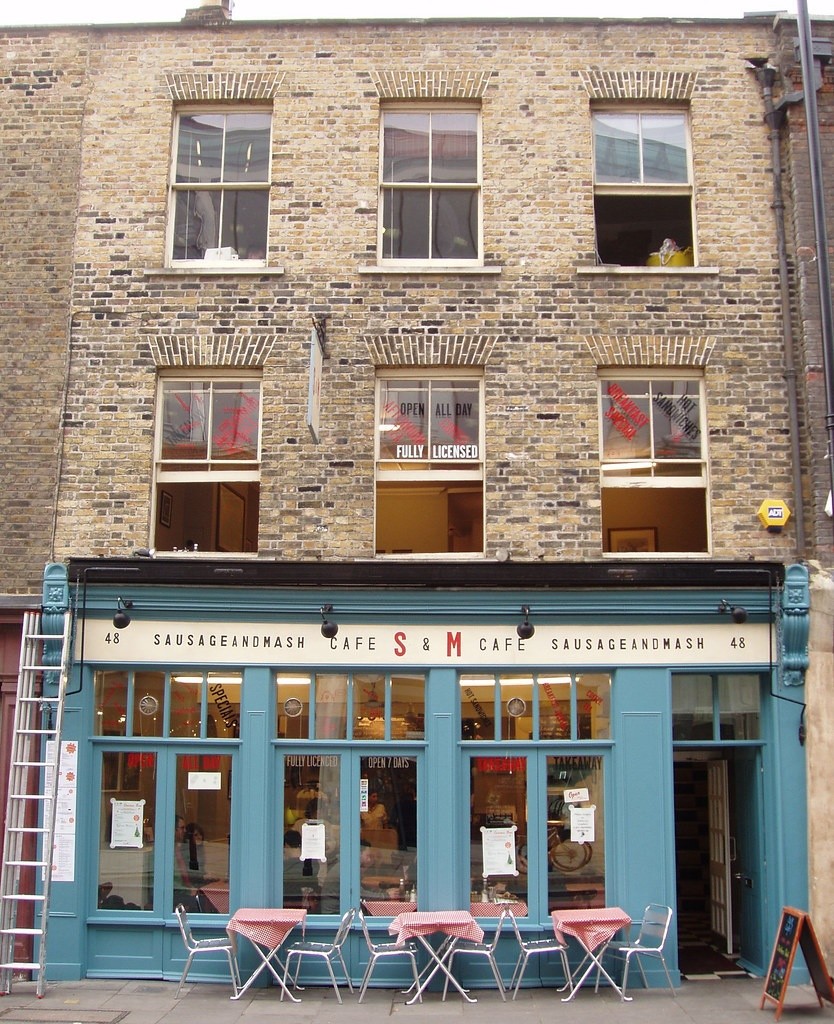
xmin=176 ymin=904 xmax=241 ymax=999
xmin=280 ymin=908 xmax=355 ymax=1003
xmin=509 ymin=908 xmax=576 ymax=1000
xmin=443 ymin=909 xmax=507 ymax=1003
xmin=596 ymin=904 xmax=680 ymax=1002
xmin=358 ymin=910 xmax=422 ymax=1004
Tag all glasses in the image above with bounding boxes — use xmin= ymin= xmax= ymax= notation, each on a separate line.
xmin=177 ymin=827 xmax=184 ymax=829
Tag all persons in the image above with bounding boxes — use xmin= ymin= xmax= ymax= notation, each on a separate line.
xmin=148 ymin=816 xmax=205 ymax=913
xmin=282 ymin=788 xmax=400 ymax=914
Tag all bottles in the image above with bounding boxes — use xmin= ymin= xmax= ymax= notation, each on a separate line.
xmin=482 ymin=879 xmax=489 ymax=903
xmin=405 ymin=891 xmax=409 ymax=901
xmin=410 ymin=890 xmax=416 ymax=903
xmin=471 ymin=892 xmax=481 ymax=902
xmin=398 ymin=879 xmax=405 ymax=901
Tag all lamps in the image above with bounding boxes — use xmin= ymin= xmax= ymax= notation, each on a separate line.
xmin=112 ymin=597 xmax=133 ymax=629
xmin=318 ymin=603 xmax=339 ymax=638
xmin=717 ymin=599 xmax=749 ymax=625
xmin=518 ymin=604 xmax=535 ymax=640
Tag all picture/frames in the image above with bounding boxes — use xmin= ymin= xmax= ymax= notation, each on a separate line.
xmin=215 ymin=483 xmax=253 ymax=553
xmin=608 ymin=526 xmax=658 ymax=554
xmin=159 ymin=491 xmax=172 ymax=528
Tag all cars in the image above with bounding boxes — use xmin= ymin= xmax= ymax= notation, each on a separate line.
xmin=280 ymin=845 xmax=417 ymax=916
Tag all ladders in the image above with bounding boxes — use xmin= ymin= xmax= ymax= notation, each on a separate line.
xmin=0 ymin=612 xmax=73 ymax=1001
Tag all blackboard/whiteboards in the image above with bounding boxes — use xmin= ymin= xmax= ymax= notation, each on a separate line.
xmin=758 ymin=906 xmax=834 ymax=1007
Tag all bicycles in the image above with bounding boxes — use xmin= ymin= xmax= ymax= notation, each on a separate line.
xmin=515 ymin=823 xmax=593 ymax=875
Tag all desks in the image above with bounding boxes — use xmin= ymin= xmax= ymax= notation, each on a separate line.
xmin=362 ymin=900 xmax=417 ymax=917
xmin=226 ymin=908 xmax=307 ymax=1002
xmin=551 ymin=908 xmax=636 ymax=1004
xmin=390 ymin=910 xmax=485 ymax=1006
xmin=470 ymin=897 xmax=529 ymax=918
xmin=201 ymin=880 xmax=229 ymax=917
